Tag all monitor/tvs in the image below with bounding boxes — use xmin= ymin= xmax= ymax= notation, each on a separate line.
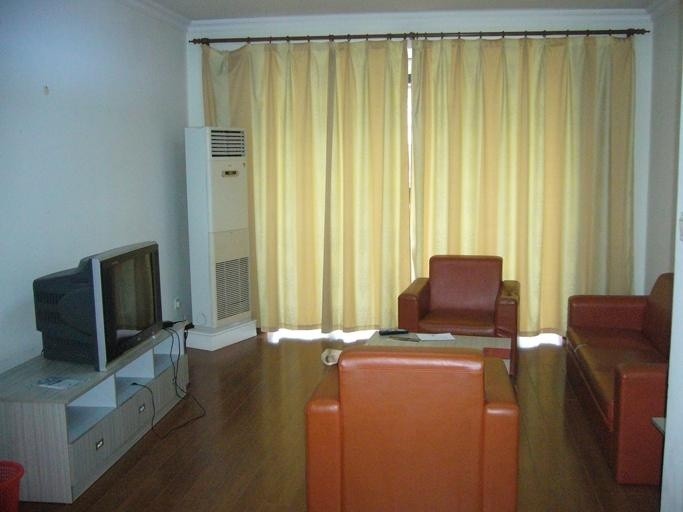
xmin=33 ymin=240 xmax=163 ymax=373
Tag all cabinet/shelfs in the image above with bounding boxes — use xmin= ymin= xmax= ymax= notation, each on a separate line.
xmin=0 ymin=322 xmax=190 ymax=504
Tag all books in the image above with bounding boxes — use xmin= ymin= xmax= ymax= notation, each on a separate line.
xmin=33 ymin=375 xmax=83 ymax=392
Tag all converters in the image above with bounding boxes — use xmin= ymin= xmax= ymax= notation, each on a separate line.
xmin=163 ymin=320 xmax=173 ymax=328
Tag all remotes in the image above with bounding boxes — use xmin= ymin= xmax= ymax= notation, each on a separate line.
xmin=379 ymin=328 xmax=409 ymax=336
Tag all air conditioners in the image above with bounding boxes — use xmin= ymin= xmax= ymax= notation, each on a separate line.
xmin=186 ymin=126 xmax=257 ymax=351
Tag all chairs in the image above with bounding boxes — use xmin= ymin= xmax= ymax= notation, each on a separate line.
xmin=398 ymin=255 xmax=520 ymax=338
xmin=304 ymin=350 xmax=519 ymax=512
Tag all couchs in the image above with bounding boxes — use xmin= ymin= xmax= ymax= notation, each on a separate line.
xmin=568 ymin=273 xmax=674 ymax=485
